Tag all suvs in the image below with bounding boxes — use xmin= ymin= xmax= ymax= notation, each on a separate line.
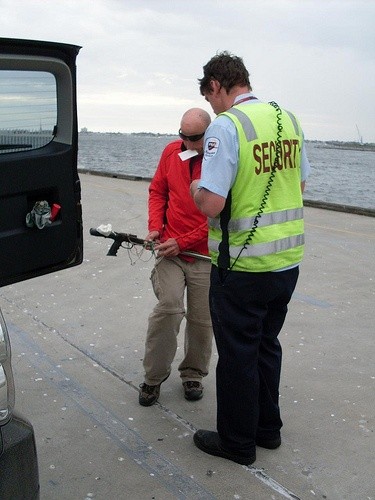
xmin=0 ymin=37 xmax=84 ymax=500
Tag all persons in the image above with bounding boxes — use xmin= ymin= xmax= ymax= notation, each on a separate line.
xmin=190 ymin=49 xmax=312 ymax=466
xmin=138 ymin=108 xmax=214 ymax=407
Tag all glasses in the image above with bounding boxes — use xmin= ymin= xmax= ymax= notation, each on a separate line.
xmin=179 ymin=128 xmax=205 ymax=141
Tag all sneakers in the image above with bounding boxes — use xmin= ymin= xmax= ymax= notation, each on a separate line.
xmin=183 ymin=381 xmax=204 ymax=401
xmin=139 ymin=372 xmax=171 ymax=407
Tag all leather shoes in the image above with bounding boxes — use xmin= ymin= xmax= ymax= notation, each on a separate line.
xmin=193 ymin=429 xmax=257 ymax=465
xmin=254 ymin=430 xmax=281 ymax=449
